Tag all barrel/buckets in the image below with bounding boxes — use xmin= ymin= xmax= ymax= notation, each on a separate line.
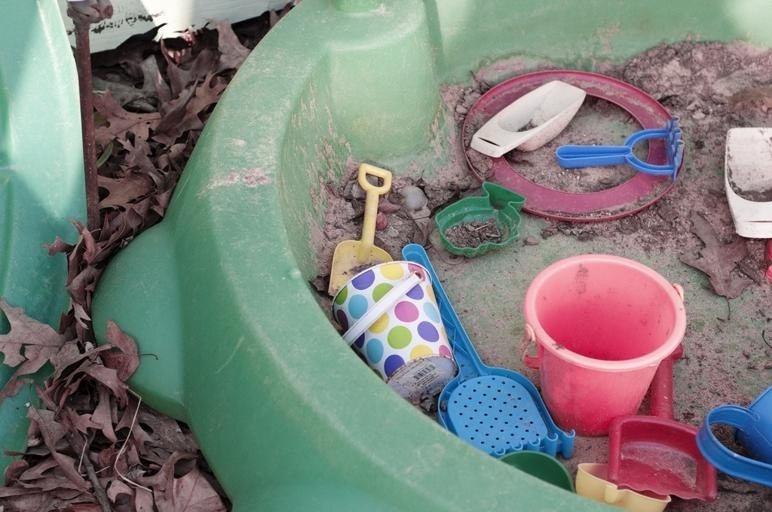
xmin=331 ymin=262 xmax=458 ymax=407
xmin=521 ymin=253 xmax=686 ymax=436
